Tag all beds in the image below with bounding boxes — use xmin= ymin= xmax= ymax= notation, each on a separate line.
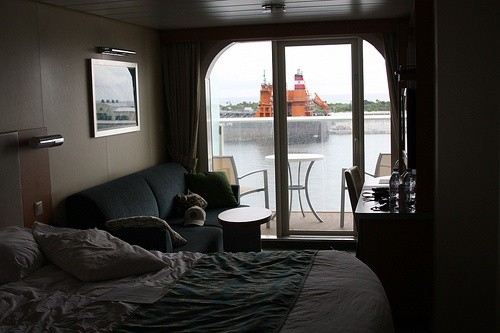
xmin=0 ymin=251 xmax=392 ymax=333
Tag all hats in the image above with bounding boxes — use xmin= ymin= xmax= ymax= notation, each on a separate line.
xmin=184 ymin=206 xmax=206 ymax=226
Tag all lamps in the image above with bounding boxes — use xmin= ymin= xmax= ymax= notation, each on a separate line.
xmin=262 ymin=0 xmax=286 ymax=15
xmin=30 ymin=134 xmax=64 ymax=149
xmin=96 ymin=46 xmax=137 ymax=57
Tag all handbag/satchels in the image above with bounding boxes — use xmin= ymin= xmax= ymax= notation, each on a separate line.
xmin=178 ymin=190 xmax=208 ymax=210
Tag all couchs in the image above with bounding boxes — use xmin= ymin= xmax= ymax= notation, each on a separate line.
xmin=65 ymin=161 xmax=239 ymax=252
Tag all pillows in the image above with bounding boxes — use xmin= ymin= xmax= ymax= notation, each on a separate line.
xmin=0 ymin=225 xmax=45 ymax=285
xmin=106 ymin=216 xmax=187 ymax=243
xmin=185 ymin=172 xmax=238 ymax=209
xmin=33 ymin=221 xmax=168 ymax=282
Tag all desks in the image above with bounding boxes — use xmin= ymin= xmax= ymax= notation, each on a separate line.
xmin=265 ymin=154 xmax=325 ymax=223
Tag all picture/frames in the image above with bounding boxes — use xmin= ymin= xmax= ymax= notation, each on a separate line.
xmin=91 ymin=58 xmax=140 ymax=138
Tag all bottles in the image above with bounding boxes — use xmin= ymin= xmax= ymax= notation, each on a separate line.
xmin=389 ymin=167 xmax=402 ymax=200
xmin=402 ymin=168 xmax=416 ymax=204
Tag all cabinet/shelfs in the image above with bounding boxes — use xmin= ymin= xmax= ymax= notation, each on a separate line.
xmin=354 ymin=178 xmax=434 ymax=333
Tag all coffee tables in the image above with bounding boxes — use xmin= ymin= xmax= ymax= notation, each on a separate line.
xmin=217 ymin=207 xmax=272 ymax=252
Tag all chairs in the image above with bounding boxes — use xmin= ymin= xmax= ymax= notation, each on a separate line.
xmin=340 ymin=152 xmax=391 ymax=227
xmin=345 ymin=166 xmax=363 ymax=212
xmin=212 ymin=156 xmax=270 ymax=229
